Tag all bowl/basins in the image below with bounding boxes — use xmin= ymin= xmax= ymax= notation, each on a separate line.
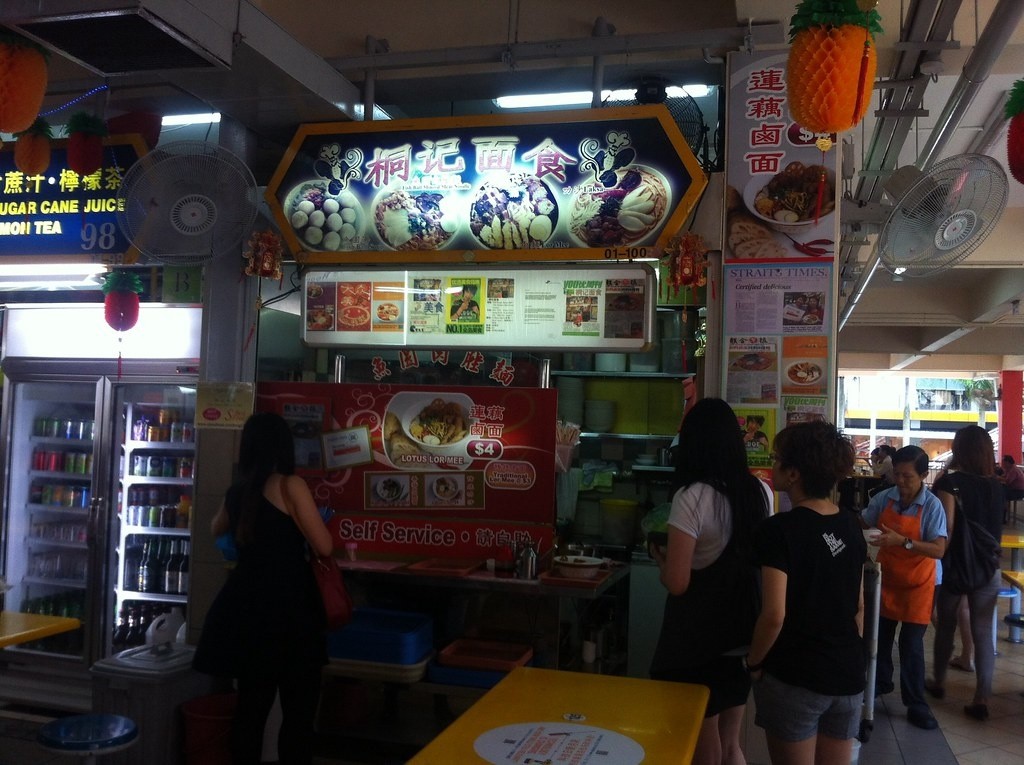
xmin=563 ymin=351 xmax=659 ymax=371
xmin=282 ymin=178 xmax=366 ymax=254
xmin=401 ymin=397 xmax=476 ymax=459
xmin=553 ymin=556 xmax=604 ymax=579
xmin=863 ymin=527 xmax=883 ymax=543
xmin=305 ymin=286 xmax=400 ymax=331
xmin=565 ymin=164 xmax=673 ymax=249
xmin=466 ymin=173 xmax=560 ymax=251
xmin=785 ymin=361 xmax=823 ymax=387
xmin=634 ymin=453 xmax=658 ymax=466
xmin=742 ymin=172 xmax=835 ymax=235
xmin=369 ymin=184 xmax=463 ymax=250
xmin=553 ymin=376 xmax=617 ymax=432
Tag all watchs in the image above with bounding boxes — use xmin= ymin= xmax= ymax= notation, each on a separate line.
xmin=741 ymin=654 xmax=763 ymax=672
xmin=903 ymin=538 xmax=913 ymax=550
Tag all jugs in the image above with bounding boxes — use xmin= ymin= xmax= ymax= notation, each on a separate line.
xmin=657 ymin=445 xmax=672 ymax=466
xmin=514 ymin=540 xmax=557 ymax=581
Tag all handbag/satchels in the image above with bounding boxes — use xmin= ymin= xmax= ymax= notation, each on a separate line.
xmin=317 ymin=557 xmax=348 ymax=617
xmin=939 ymin=475 xmax=1001 ymax=593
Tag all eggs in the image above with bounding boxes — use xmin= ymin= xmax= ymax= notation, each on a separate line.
xmin=441 ymin=213 xmax=458 ymax=232
xmin=774 ymin=210 xmax=801 ymax=223
xmin=423 ymin=435 xmax=441 ymax=445
xmin=529 ymin=214 xmax=553 ymax=242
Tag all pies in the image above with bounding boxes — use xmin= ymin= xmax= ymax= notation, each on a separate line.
xmin=383 ymin=410 xmax=420 ymax=468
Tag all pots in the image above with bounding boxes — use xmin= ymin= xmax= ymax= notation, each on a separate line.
xmin=550 ymin=536 xmax=627 ymax=557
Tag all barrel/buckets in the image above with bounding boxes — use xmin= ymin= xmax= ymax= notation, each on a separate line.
xmin=599 ymin=499 xmax=639 ymax=544
xmin=661 ymin=311 xmax=698 ymax=373
xmin=585 ymin=628 xmax=611 ymax=657
xmin=180 ymin=694 xmax=239 ymax=765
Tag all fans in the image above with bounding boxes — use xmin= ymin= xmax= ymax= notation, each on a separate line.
xmin=114 ymin=139 xmax=260 ymax=270
xmin=876 ymin=148 xmax=1007 ymax=279
xmin=599 ymin=74 xmax=706 ymax=157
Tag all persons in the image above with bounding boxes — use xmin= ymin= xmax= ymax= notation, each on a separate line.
xmin=743 ymin=421 xmax=867 ymax=765
xmin=924 ymin=425 xmax=1001 ymax=720
xmin=192 ymin=412 xmax=332 ymax=765
xmin=580 ymin=296 xmax=591 ymax=322
xmin=451 ymin=284 xmax=479 ymax=319
xmin=857 ymin=446 xmax=949 ymax=729
xmin=740 ymin=415 xmax=769 ymax=447
xmin=864 ymin=444 xmax=896 ymax=499
xmin=999 ymin=455 xmax=1024 ymax=523
xmin=648 ymin=398 xmax=774 ymax=765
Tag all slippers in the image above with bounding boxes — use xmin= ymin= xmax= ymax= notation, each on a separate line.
xmin=948 ymin=655 xmax=972 ymax=672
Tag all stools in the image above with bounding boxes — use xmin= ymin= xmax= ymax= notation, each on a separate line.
xmin=35 ymin=713 xmax=140 ymax=765
xmin=991 ymin=589 xmax=1024 ymax=655
xmin=1008 ymin=496 xmax=1022 ymax=528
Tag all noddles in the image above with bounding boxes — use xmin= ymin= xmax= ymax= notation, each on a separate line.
xmin=754 ymin=176 xmax=833 ymax=223
xmin=375 ymin=192 xmax=452 ymax=251
xmin=570 ymin=167 xmax=666 ymax=247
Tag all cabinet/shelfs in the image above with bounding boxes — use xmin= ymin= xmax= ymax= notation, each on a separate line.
xmin=544 ymin=370 xmax=695 ymax=555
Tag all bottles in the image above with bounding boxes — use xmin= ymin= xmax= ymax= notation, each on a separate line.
xmin=18 ymin=384 xmax=197 ymax=663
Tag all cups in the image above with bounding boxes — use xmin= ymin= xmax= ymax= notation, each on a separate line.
xmin=556 ymin=445 xmax=572 ymax=473
xmin=583 ymin=641 xmax=596 ymax=663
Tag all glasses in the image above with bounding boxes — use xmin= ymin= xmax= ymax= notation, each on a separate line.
xmin=769 ymin=453 xmax=786 ymax=465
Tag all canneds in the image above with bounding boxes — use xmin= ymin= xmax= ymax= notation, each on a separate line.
xmin=127 ymin=409 xmax=193 ymax=528
xmin=29 ymin=416 xmax=95 ymax=542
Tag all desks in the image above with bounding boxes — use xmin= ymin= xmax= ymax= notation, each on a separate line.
xmin=999 ymin=536 xmax=1024 ymax=645
xmin=0 ymin=609 xmax=81 ymax=647
xmin=1000 ymin=570 xmax=1024 ymax=592
xmin=399 ymin=665 xmax=711 ymax=765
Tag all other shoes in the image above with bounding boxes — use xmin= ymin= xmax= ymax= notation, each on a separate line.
xmin=964 ymin=704 xmax=989 ymax=721
xmin=864 ymin=683 xmax=894 ymax=703
xmin=925 ymin=677 xmax=945 ymax=698
xmin=906 ymin=708 xmax=938 ymax=730
